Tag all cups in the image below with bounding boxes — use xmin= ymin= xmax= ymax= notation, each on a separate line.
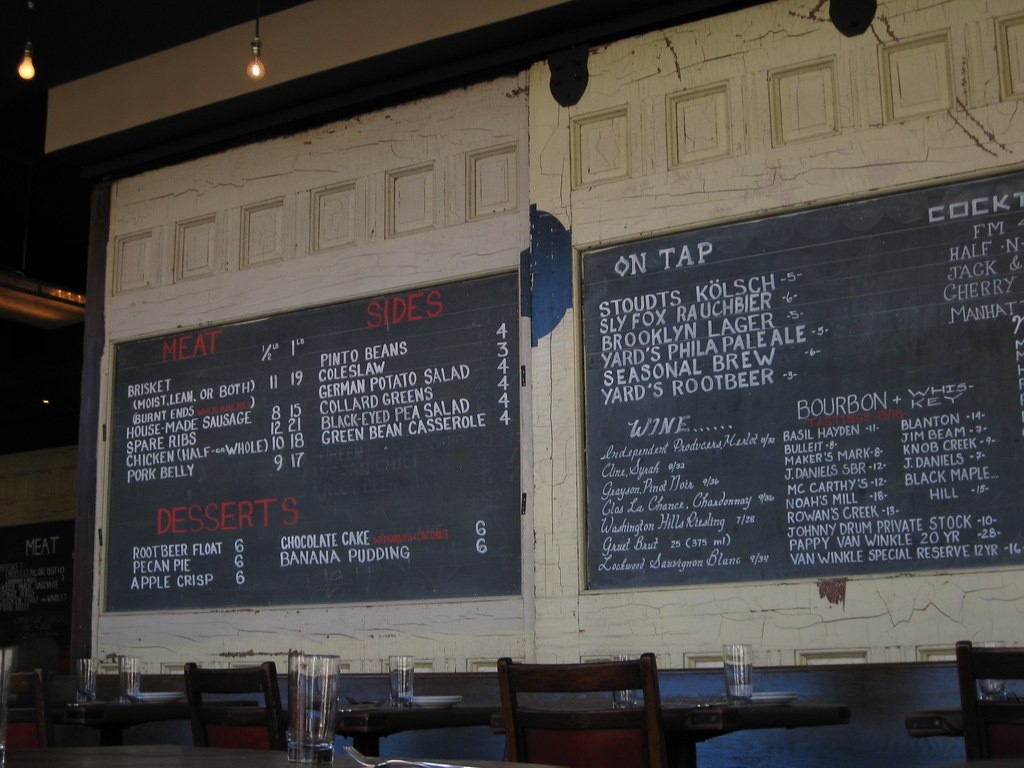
xmin=612 ymin=655 xmax=639 ymax=708
xmin=287 ymin=653 xmax=340 ymax=765
xmin=118 ymin=656 xmax=141 ymax=704
xmin=389 ymin=656 xmax=415 ymax=708
xmin=972 ymin=641 xmax=1007 ymax=700
xmin=722 ymin=643 xmax=753 ymax=698
xmin=0 ymin=648 xmax=16 ymax=768
xmin=75 ymin=659 xmax=97 ymax=703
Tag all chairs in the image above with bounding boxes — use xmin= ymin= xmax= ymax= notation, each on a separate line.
xmin=497 ymin=652 xmax=664 ymax=768
xmin=183 ymin=661 xmax=288 ymax=752
xmin=955 ymin=641 xmax=1024 ymax=768
xmin=9 ymin=667 xmax=53 ymax=748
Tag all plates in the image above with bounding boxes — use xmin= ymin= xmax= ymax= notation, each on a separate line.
xmin=751 ymin=692 xmax=797 ymax=703
xmin=140 ymin=692 xmax=184 ymax=703
xmin=413 ymin=696 xmax=463 ymax=708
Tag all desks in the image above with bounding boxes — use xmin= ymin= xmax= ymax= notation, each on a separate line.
xmin=906 ymin=709 xmax=1024 ymax=768
xmin=5 ymin=746 xmax=563 ymax=768
xmin=230 ymin=700 xmax=502 ymax=758
xmin=5 ymin=698 xmax=257 ymax=745
xmin=493 ymin=696 xmax=851 ymax=767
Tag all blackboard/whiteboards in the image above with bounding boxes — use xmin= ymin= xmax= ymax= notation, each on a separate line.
xmin=572 ymin=158 xmax=1024 ymax=583
xmin=103 ymin=264 xmax=521 ymax=604
xmin=0 ymin=519 xmax=75 ymax=672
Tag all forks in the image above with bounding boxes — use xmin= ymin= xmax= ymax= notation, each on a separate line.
xmin=342 ymin=745 xmax=478 ymax=768
xmin=1007 ymin=691 xmax=1024 ymax=703
